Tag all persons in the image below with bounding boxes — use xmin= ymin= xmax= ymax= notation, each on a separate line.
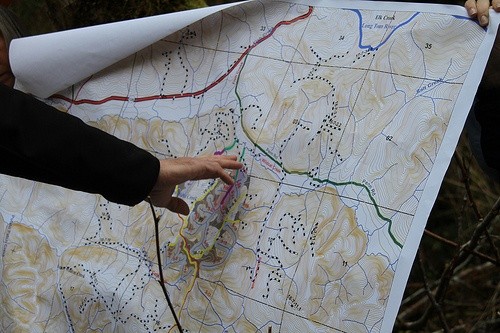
xmin=0 ymin=2 xmax=243 ymax=215
